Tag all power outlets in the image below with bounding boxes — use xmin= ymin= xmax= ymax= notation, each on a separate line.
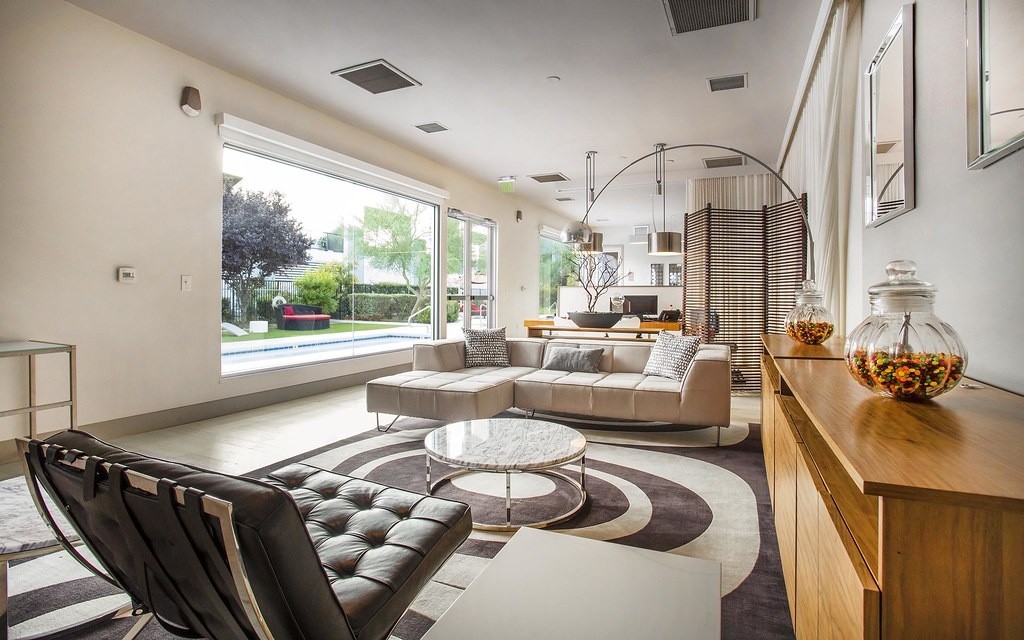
xmin=181 ymin=275 xmax=192 ymax=292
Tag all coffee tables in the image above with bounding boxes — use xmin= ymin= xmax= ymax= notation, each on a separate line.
xmin=423 ymin=418 xmax=588 ymax=533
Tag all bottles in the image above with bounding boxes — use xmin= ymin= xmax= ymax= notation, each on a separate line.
xmin=843 ymin=259 xmax=970 ymax=403
xmin=783 ymin=280 xmax=836 ymax=345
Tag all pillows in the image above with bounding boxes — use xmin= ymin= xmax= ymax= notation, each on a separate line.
xmin=282 ymin=305 xmax=295 ymax=315
xmin=540 ymin=347 xmax=605 ymax=374
xmin=460 ymin=326 xmax=512 ymax=368
xmin=641 ymin=329 xmax=704 ymax=383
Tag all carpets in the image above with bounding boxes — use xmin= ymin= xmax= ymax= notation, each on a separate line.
xmin=0 ymin=417 xmax=797 ymax=640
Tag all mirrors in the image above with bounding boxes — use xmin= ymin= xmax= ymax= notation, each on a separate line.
xmin=865 ymin=2 xmax=916 ymax=229
xmin=967 ymin=0 xmax=1024 ymax=171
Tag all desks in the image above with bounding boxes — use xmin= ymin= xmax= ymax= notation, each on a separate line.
xmin=524 ymin=318 xmax=683 ymax=339
xmin=0 ymin=340 xmax=76 ymax=441
xmin=420 ymin=525 xmax=722 ymax=640
xmin=283 ymin=314 xmax=331 ymax=331
xmin=527 ymin=326 xmax=664 ymax=342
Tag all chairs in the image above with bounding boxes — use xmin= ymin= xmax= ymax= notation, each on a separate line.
xmin=14 ymin=429 xmax=473 ymax=640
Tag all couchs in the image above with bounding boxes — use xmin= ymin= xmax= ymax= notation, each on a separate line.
xmin=471 ymin=304 xmax=487 ymax=319
xmin=273 ymin=303 xmax=323 ymax=330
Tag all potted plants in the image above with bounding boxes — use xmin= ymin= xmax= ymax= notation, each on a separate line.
xmin=560 ymin=249 xmax=632 ymax=329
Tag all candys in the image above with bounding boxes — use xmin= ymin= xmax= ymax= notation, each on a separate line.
xmin=845 ymin=349 xmax=963 ymax=399
xmin=784 ymin=321 xmax=835 ymax=345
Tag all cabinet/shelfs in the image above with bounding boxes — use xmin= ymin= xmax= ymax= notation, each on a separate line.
xmin=760 ymin=333 xmax=1024 ymax=640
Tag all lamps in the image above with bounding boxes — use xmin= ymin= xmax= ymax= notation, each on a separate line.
xmin=560 ymin=144 xmax=817 ymax=283
xmin=181 ymin=86 xmax=201 ymax=118
xmin=648 ymin=143 xmax=681 ymax=256
xmin=575 ymin=151 xmax=603 ymax=253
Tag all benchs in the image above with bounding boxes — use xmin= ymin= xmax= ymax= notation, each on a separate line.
xmin=367 ymin=337 xmax=731 ymax=448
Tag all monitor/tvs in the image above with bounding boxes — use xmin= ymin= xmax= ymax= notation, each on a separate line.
xmin=622 ymin=295 xmax=660 ymax=321
